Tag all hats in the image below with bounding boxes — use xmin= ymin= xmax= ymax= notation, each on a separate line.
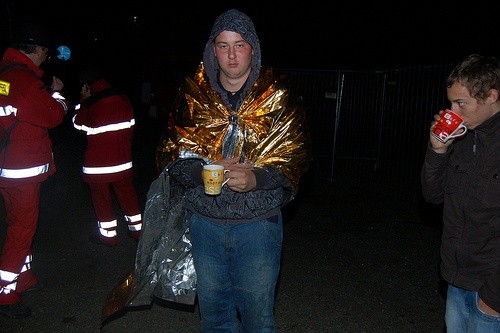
xmin=11 ymin=38 xmax=60 ymax=56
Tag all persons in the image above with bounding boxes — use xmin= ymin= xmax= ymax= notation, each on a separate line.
xmin=0 ymin=35 xmax=69 ymax=308
xmin=155 ymin=10 xmax=307 ymax=331
xmin=72 ymin=79 xmax=143 ymax=246
xmin=420 ymin=58 xmax=500 ymax=333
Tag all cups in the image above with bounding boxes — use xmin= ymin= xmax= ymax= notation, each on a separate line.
xmin=203 ymin=165 xmax=231 ymax=195
xmin=432 ymin=109 xmax=467 ymax=145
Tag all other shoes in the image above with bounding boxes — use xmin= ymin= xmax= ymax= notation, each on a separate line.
xmin=21 ymin=279 xmax=48 ymax=294
xmin=127 ymin=231 xmax=140 ymax=242
xmin=0 ymin=302 xmax=31 ymax=319
xmin=88 ymin=235 xmax=118 ymax=247
